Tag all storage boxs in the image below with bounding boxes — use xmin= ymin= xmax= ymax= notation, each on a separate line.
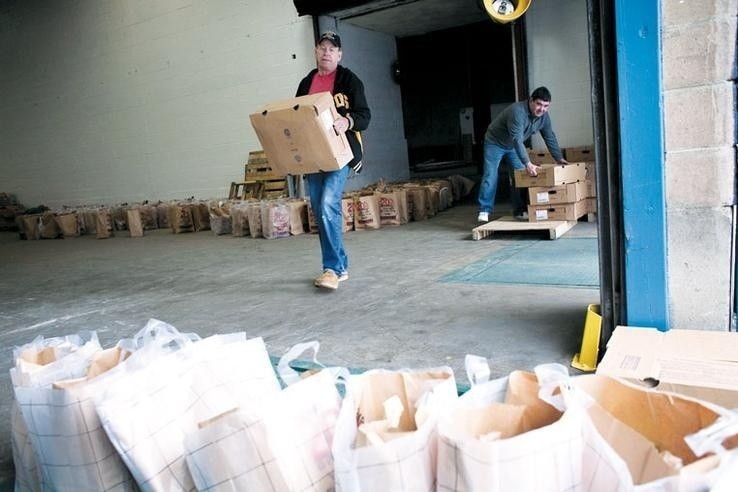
xmin=594 ymin=325 xmax=738 ymax=411
xmin=514 ymin=146 xmax=597 ymax=222
xmin=249 ymin=91 xmax=355 ymax=176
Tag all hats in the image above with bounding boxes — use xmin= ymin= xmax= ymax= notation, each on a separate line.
xmin=317 ymin=30 xmax=342 ymax=48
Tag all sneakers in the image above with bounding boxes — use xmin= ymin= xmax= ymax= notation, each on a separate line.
xmin=477 ymin=212 xmax=490 ymax=223
xmin=514 ymin=211 xmax=529 ymax=220
xmin=314 ymin=268 xmax=348 ymax=291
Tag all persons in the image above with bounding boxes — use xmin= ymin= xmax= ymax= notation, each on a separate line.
xmin=293 ymin=32 xmax=371 ymax=290
xmin=476 ymin=85 xmax=569 ymax=224
